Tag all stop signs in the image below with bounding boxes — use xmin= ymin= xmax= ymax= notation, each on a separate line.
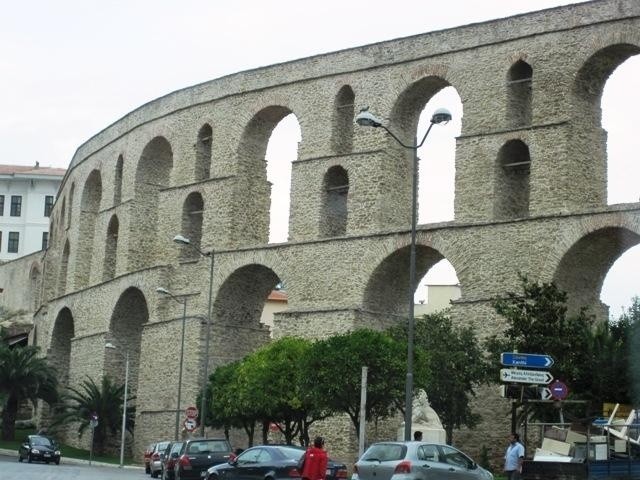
xmin=184 ymin=405 xmax=199 ymax=419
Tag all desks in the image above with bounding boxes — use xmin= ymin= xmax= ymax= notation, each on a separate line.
xmin=602 ymin=403 xmax=640 ymax=448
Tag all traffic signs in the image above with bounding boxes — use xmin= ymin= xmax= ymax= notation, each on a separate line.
xmin=502 ymin=352 xmax=556 ymax=369
xmin=497 ymin=369 xmax=555 ymax=386
xmin=498 ymin=384 xmax=553 ymax=402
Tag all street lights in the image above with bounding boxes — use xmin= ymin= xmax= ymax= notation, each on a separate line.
xmin=99 ymin=340 xmax=134 ymax=468
xmin=153 ymin=286 xmax=190 ymax=442
xmin=351 ymin=102 xmax=452 ymax=434
xmin=171 ymin=234 xmax=216 ymax=439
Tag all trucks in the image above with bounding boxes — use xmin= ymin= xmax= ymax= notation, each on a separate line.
xmin=518 ymin=398 xmax=640 ymax=480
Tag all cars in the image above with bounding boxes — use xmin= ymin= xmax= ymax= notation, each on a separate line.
xmin=17 ymin=434 xmax=63 ymax=468
xmin=204 ymin=444 xmax=347 ymax=480
xmin=351 ymin=440 xmax=495 ymax=480
xmin=143 ymin=437 xmax=237 ymax=480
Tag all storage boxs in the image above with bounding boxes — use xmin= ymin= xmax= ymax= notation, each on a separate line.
xmin=527 ymin=418 xmax=628 ymax=464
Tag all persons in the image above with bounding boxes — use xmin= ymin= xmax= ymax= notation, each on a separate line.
xmin=504 ymin=433 xmax=525 ymax=480
xmin=297 ymin=436 xmax=328 ymax=480
xmin=413 ymin=430 xmax=422 ymax=441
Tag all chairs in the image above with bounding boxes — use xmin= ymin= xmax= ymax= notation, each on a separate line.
xmin=189 ymin=446 xmax=201 ymax=455
xmin=212 ymin=443 xmax=224 ymax=453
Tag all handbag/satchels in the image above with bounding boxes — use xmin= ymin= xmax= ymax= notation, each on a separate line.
xmin=296 ymin=454 xmax=305 ymax=471
